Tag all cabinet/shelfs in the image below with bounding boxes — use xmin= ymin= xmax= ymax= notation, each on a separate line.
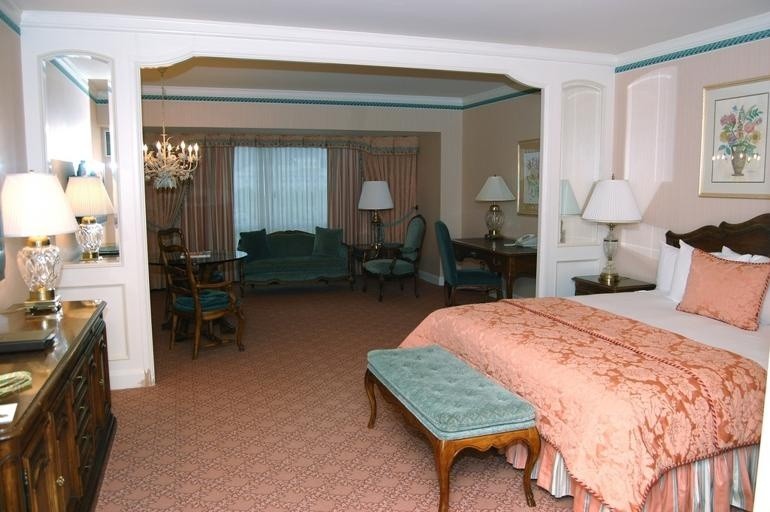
xmin=0 ymin=299 xmax=117 ymax=512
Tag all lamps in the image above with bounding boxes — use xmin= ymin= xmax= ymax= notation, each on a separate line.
xmin=63 ymin=178 xmax=115 ymax=261
xmin=358 ymin=181 xmax=394 ymax=249
xmin=582 ymin=174 xmax=641 ymax=283
xmin=143 ymin=67 xmax=201 ymax=190
xmin=1 ymin=173 xmax=79 ymax=315
xmin=475 ymin=174 xmax=516 ymax=240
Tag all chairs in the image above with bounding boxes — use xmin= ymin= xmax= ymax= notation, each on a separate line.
xmin=362 ymin=215 xmax=426 ymax=301
xmin=434 ymin=221 xmax=503 ymax=306
xmin=159 ymin=244 xmax=247 ymax=360
xmin=156 ymin=229 xmax=224 ymax=328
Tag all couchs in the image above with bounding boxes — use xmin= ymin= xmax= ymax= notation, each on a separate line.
xmin=238 ymin=227 xmax=355 ymax=297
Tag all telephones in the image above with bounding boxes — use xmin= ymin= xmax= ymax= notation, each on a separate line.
xmin=504 ymin=232 xmax=537 ymax=249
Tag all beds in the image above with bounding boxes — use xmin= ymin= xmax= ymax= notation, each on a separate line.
xmin=394 ymin=214 xmax=770 ymax=512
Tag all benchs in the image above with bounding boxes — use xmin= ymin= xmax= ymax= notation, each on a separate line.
xmin=364 ymin=344 xmax=542 ymax=512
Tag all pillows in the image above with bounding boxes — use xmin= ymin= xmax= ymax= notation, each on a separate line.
xmin=656 ymin=242 xmax=680 ymax=297
xmin=676 ymin=248 xmax=770 ymax=332
xmin=240 ymin=229 xmax=271 ymax=263
xmin=312 ymin=226 xmax=343 ymax=259
xmin=668 ymin=239 xmax=753 ymax=305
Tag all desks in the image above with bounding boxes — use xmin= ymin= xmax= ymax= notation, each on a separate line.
xmin=452 ymin=236 xmax=538 ymax=298
xmin=149 ymin=249 xmax=248 ymax=329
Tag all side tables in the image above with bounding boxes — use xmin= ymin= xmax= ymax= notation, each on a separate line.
xmin=350 ymin=244 xmax=398 ymax=262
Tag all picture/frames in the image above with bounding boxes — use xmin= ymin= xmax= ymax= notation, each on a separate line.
xmin=699 ymin=76 xmax=769 ymax=199
xmin=516 ymin=140 xmax=540 ymax=215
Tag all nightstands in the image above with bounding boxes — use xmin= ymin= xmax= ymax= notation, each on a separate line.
xmin=571 ymin=273 xmax=657 ymax=296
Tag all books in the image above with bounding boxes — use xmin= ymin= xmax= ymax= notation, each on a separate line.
xmin=180 ymin=251 xmax=211 ymax=258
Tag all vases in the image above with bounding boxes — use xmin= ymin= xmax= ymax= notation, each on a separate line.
xmin=730 ymin=151 xmax=746 ymax=176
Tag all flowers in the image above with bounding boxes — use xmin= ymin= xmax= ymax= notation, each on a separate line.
xmin=713 ymin=104 xmax=763 ymax=161
